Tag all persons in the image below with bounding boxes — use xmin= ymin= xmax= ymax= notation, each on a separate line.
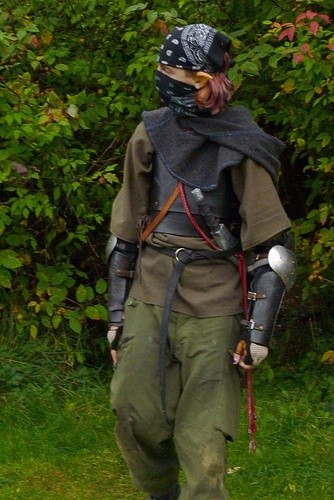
xmin=104 ymin=24 xmax=297 ymax=499
